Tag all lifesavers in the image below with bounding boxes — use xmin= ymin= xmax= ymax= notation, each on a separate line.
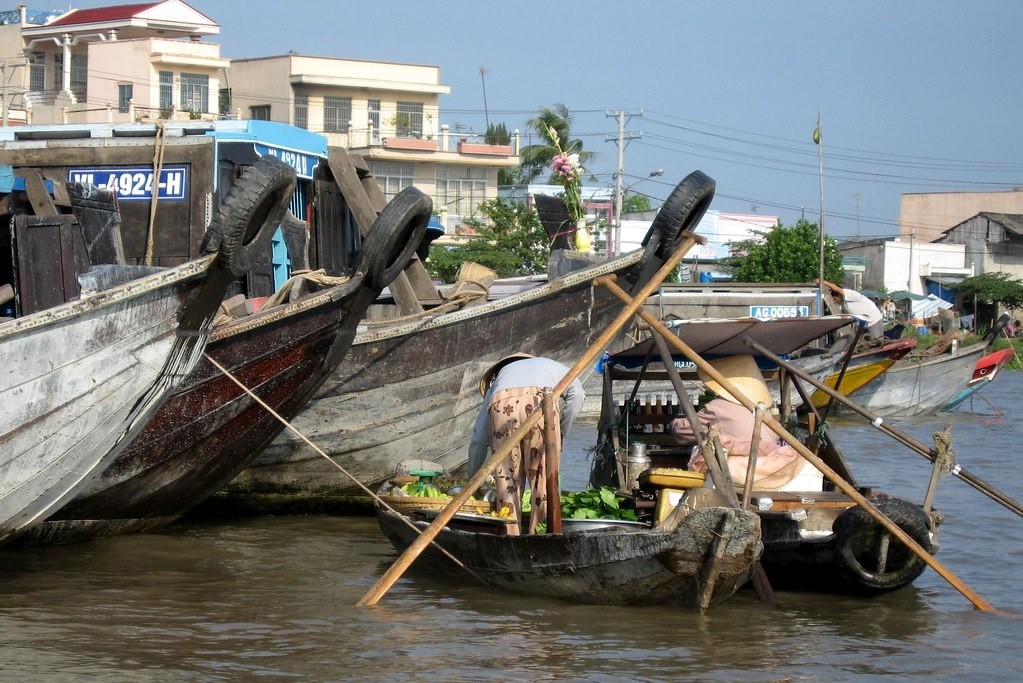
xmin=350 ymin=185 xmax=433 ymax=292
xmin=641 ymin=168 xmax=717 ymax=263
xmin=835 ymin=497 xmax=935 ymax=591
xmin=200 ymin=153 xmax=301 ymax=283
xmin=983 ymin=314 xmax=1010 ymax=347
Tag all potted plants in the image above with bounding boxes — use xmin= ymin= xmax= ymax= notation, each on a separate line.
xmin=382 ymin=115 xmax=438 ymax=152
xmin=457 ymin=122 xmax=513 ymax=156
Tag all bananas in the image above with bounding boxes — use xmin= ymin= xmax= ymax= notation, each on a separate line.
xmin=385 ymin=482 xmax=513 ymax=520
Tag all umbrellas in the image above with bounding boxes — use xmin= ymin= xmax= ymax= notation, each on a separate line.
xmin=860 ymin=290 xmax=937 ymax=302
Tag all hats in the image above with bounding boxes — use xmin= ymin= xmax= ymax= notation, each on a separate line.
xmin=697 ymin=355 xmax=773 ymax=409
xmin=479 ymin=351 xmax=535 ymax=399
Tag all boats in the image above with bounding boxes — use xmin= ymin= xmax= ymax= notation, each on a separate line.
xmin=587 ymin=314 xmax=951 ymax=594
xmin=193 ymin=171 xmax=715 ymax=515
xmin=1 ymin=156 xmax=296 ymax=549
xmin=573 ymin=313 xmax=1015 ymax=428
xmin=372 ymin=461 xmax=764 ymax=616
xmin=9 ymin=184 xmax=433 ymax=546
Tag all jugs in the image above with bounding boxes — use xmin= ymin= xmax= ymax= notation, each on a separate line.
xmin=615 ymin=442 xmax=652 ymax=495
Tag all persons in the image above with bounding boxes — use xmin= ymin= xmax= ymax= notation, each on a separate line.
xmin=467 ymin=352 xmax=586 ymax=535
xmin=815 ymin=278 xmax=884 ymax=341
xmin=671 ymin=354 xmax=781 ymax=474
xmin=1004 ymin=312 xmax=1017 ymax=336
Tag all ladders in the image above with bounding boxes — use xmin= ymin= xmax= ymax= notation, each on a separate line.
xmin=12 ymin=168 xmax=72 ymax=216
xmin=327 ymin=147 xmax=443 ymax=317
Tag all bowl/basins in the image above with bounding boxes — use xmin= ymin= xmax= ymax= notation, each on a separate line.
xmin=410 ymin=507 xmax=519 ymax=534
xmin=560 ymin=515 xmax=653 ymax=533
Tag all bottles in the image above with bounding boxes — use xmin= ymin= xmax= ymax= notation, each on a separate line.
xmin=613 ymin=399 xmax=621 ymax=429
xmin=6 ymin=308 xmax=13 ymax=317
xmin=624 ymin=393 xmax=695 ymax=416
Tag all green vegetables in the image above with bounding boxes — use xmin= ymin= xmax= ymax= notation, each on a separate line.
xmin=534 ymin=486 xmax=639 ymax=535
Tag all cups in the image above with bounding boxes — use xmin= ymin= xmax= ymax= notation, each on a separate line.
xmin=653 ymin=445 xmax=661 ymax=450
xmin=643 ymin=424 xmax=665 ymax=433
xmin=447 ymin=485 xmax=465 ymax=496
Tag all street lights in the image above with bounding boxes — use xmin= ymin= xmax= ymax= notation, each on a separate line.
xmin=608 ymin=169 xmax=664 ymax=256
